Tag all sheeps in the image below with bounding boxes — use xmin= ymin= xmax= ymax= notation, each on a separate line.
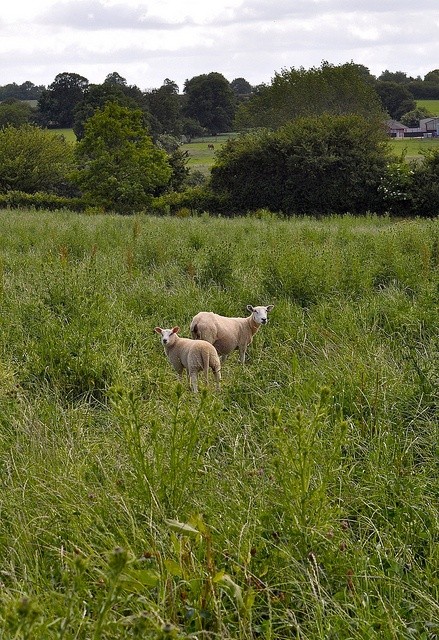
xmin=187 ymin=304 xmax=275 ymax=367
xmin=153 ymin=326 xmax=223 ymax=397
